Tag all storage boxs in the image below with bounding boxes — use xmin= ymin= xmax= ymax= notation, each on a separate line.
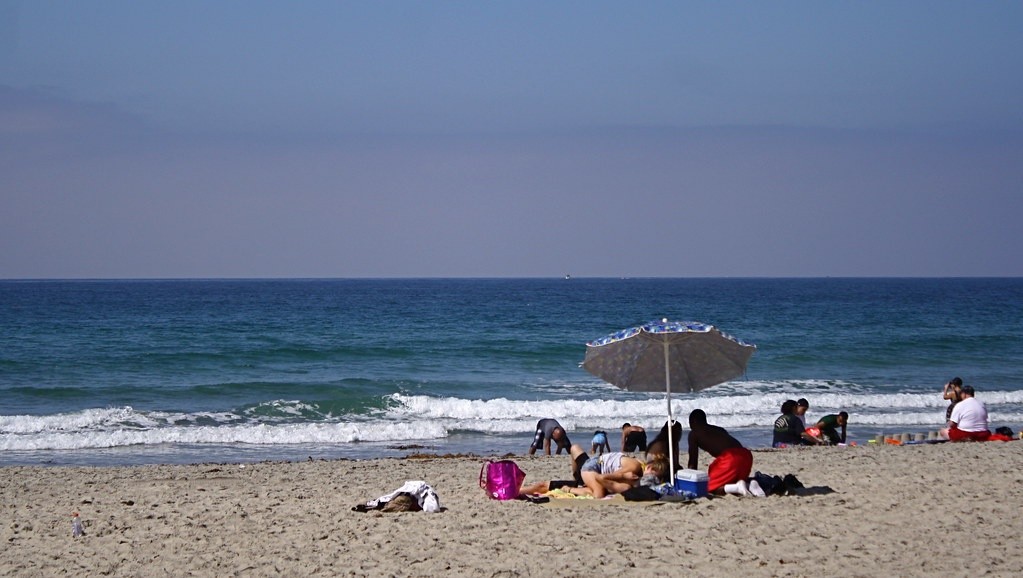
xmin=674 ymin=469 xmax=709 ymax=498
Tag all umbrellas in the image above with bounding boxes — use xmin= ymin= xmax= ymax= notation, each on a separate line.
xmin=578 ymin=318 xmax=757 ymax=487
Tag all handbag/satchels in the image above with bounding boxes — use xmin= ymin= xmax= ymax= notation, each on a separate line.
xmin=480 ymin=459 xmax=527 ymax=501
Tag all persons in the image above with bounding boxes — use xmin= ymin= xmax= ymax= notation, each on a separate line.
xmin=519 ymin=420 xmax=683 ymax=498
xmin=772 ymin=398 xmax=849 ymax=448
xmin=591 ymin=430 xmax=611 ymax=456
xmin=939 ymin=377 xmax=992 ymax=443
xmin=529 ymin=419 xmax=573 ymax=456
xmin=622 ymin=423 xmax=647 ymax=453
xmin=688 ymin=409 xmax=766 ymax=498
